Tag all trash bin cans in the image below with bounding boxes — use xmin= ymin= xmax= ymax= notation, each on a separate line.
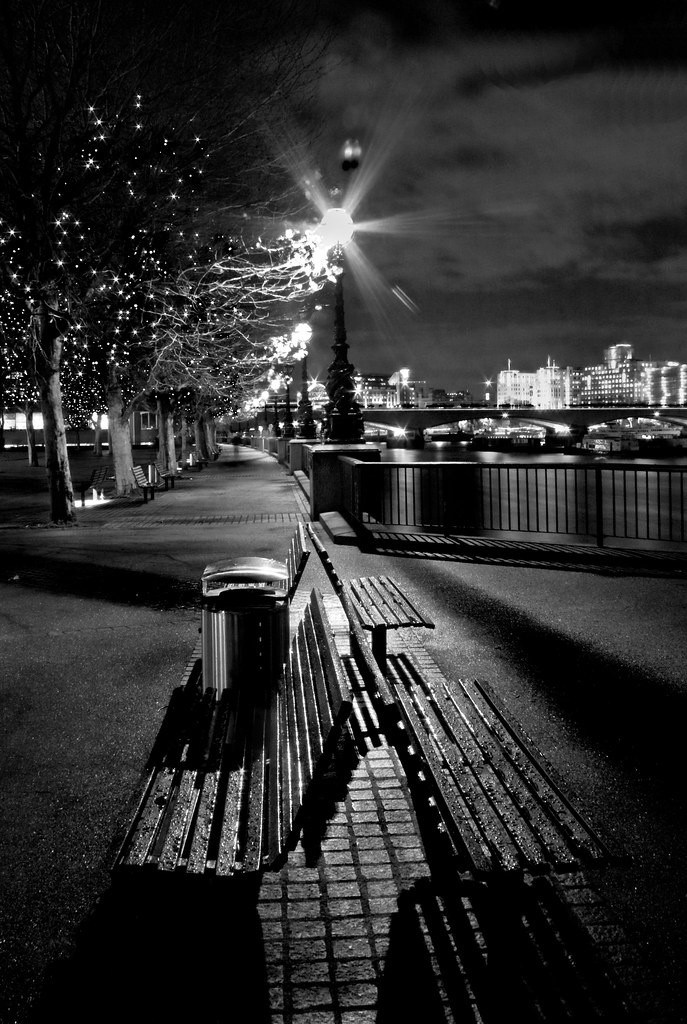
xmin=148 ymin=461 xmax=159 ymax=484
xmin=201 ymin=555 xmax=293 ymax=703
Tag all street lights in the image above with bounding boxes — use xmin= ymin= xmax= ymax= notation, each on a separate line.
xmin=318 ymin=205 xmax=368 ymax=445
xmin=268 ymin=380 xmax=283 ymax=438
xmin=292 ymin=320 xmax=319 ymax=440
xmin=243 ymin=403 xmax=252 ymax=437
xmin=261 ymin=390 xmax=271 ymax=439
xmin=253 ymin=397 xmax=262 ymax=436
xmin=275 ymin=342 xmax=296 ymax=440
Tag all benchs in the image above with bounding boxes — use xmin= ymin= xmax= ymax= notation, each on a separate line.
xmin=72 ymin=466 xmax=108 ymax=506
xmin=131 ymin=466 xmax=165 ymax=500
xmin=153 ymin=459 xmax=181 ymax=491
xmin=91 ymin=521 xmax=633 ymax=909
